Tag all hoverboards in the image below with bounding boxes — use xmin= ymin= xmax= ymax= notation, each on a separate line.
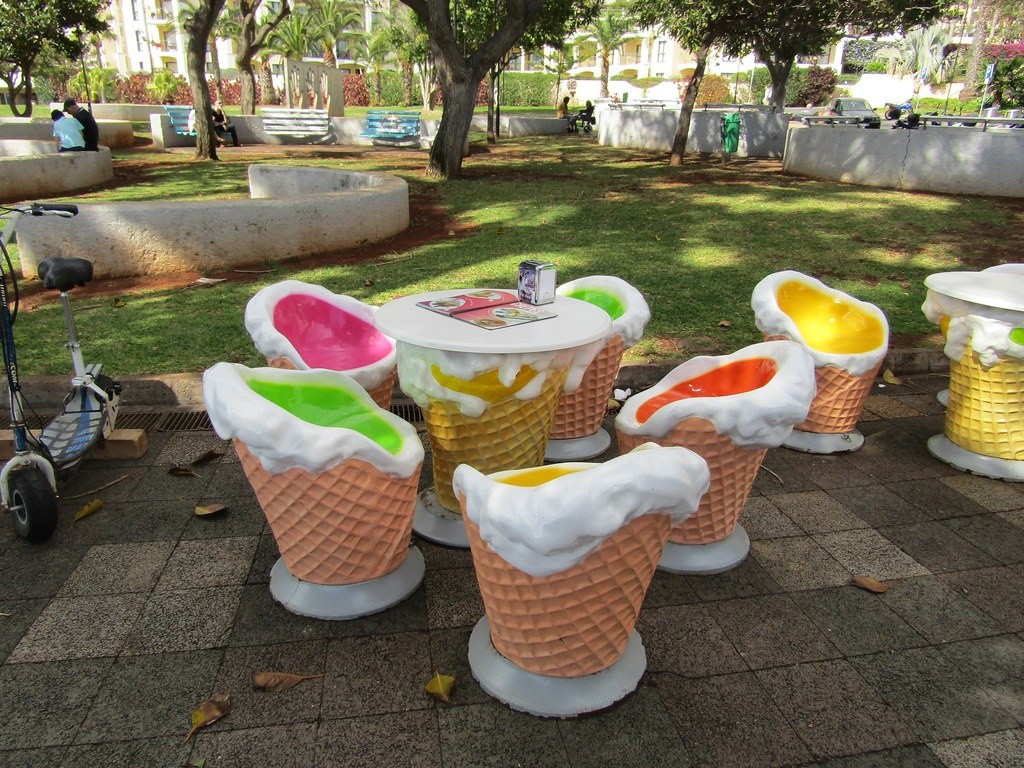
xmin=0 ymin=198 xmax=124 ymax=542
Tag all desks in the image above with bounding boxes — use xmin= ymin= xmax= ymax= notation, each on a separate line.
xmin=923 ymin=266 xmax=1024 ymax=344
xmin=376 ymin=287 xmax=616 ymax=552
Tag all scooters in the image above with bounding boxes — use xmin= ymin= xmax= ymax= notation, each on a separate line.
xmin=894 ymin=104 xmax=1024 ymax=129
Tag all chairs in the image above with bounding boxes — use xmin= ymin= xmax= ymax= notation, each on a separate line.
xmin=613 ymin=335 xmax=820 ymax=588
xmin=750 ymin=268 xmax=892 ymax=454
xmin=244 ymin=278 xmax=398 ymax=415
xmin=452 ymin=445 xmax=711 ymax=725
xmin=203 ymin=361 xmax=430 ymax=623
xmin=527 ymin=271 xmax=645 ymax=464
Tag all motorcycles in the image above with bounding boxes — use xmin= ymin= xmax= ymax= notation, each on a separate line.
xmin=885 ymin=98 xmax=914 ymax=120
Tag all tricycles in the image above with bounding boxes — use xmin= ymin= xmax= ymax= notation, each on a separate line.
xmin=569 ymin=104 xmax=596 ymax=135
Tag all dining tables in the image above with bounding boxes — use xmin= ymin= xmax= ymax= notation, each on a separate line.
xmin=924 ymin=312 xmax=1024 ymax=483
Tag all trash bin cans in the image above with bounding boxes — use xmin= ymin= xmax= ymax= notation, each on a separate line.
xmin=719 ymin=112 xmax=742 ymax=155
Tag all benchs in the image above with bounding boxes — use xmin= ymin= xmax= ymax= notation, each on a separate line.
xmin=257 ymin=105 xmax=331 ymax=137
xmin=359 ymin=108 xmax=423 ymax=144
xmin=164 ymin=104 xmax=235 ymax=138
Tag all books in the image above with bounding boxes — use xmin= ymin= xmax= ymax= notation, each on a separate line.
xmin=417 ymin=288 xmax=558 ymax=330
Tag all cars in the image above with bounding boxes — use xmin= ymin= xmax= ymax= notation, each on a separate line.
xmin=820 ymin=96 xmax=881 ymax=129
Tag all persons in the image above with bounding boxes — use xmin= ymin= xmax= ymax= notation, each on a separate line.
xmin=50 ymin=101 xmax=99 ymax=151
xmin=189 ymin=100 xmax=247 ymax=147
xmin=559 ymin=97 xmax=593 ymax=132
